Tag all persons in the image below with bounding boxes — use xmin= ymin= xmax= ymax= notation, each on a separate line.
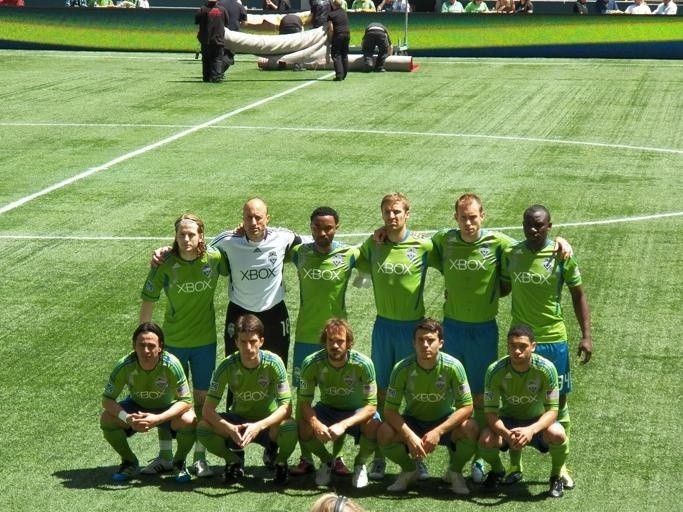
xmin=299 ymin=318 xmax=383 ymax=488
xmin=392 ymin=0 xmax=411 ymax=12
xmin=279 ymin=15 xmax=304 ymax=33
xmin=309 ymin=0 xmax=332 ymax=28
xmin=573 ymin=0 xmax=588 ymax=15
xmin=359 ymin=193 xmax=443 ymax=481
xmin=217 ymin=0 xmax=248 ymax=72
xmin=477 ymin=324 xmax=569 ymax=497
xmin=495 ymin=0 xmax=516 ymax=13
xmin=309 ymin=492 xmax=365 ymax=512
xmin=376 ymin=316 xmax=481 ymax=495
xmin=65 ymin=0 xmax=88 ymax=7
xmin=515 ymin=0 xmax=533 ymax=13
xmin=377 ymin=0 xmax=396 ymax=12
xmin=93 ymin=0 xmax=114 ymax=7
xmin=351 ymin=0 xmax=376 ymax=12
xmin=140 ymin=214 xmax=231 ymax=479
xmin=440 ymin=0 xmax=464 ymax=12
xmin=0 ymin=0 xmax=25 ymax=7
xmin=233 ymin=206 xmax=371 ymax=477
xmin=624 ymin=0 xmax=651 ymax=14
xmin=196 ymin=314 xmax=299 ymax=486
xmin=100 ymin=322 xmax=199 ymax=484
xmin=327 ymin=0 xmax=350 ymax=82
xmin=652 ymin=0 xmax=677 ymax=15
xmin=465 ymin=0 xmax=489 ymax=12
xmin=361 ymin=22 xmax=393 ymax=72
xmin=196 ymin=0 xmax=230 ymax=83
xmin=151 ymin=198 xmax=313 ymax=476
xmin=115 ymin=0 xmax=136 ymax=8
xmin=590 ymin=0 xmax=619 ymax=13
xmin=374 ymin=193 xmax=574 ymax=484
xmin=263 ymin=0 xmax=291 ymax=11
xmin=445 ymin=204 xmax=591 ymax=488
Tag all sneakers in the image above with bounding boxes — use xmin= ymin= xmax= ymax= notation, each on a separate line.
xmin=112 ymin=461 xmax=140 ymax=484
xmin=478 ymin=469 xmax=507 ymax=497
xmin=262 ymin=451 xmax=278 ymax=466
xmin=472 ymin=461 xmax=485 ymax=485
xmin=224 ymin=461 xmax=246 ymax=485
xmin=504 ymin=470 xmax=523 ymax=485
xmin=333 ymin=455 xmax=351 ymax=477
xmin=169 ymin=457 xmax=193 ymax=482
xmin=140 ymin=457 xmax=171 ymax=477
xmin=367 ymin=459 xmax=387 ymax=481
xmin=351 ymin=465 xmax=370 ymax=489
xmin=561 ymin=470 xmax=574 ymax=489
xmin=192 ymin=458 xmax=215 ymax=479
xmin=274 ymin=458 xmax=291 ymax=490
xmin=386 ymin=468 xmax=422 ymax=492
xmin=441 ymin=464 xmax=471 ymax=496
xmin=549 ymin=476 xmax=564 ymax=498
xmin=417 ymin=460 xmax=433 ymax=480
xmin=290 ymin=456 xmax=317 ymax=476
xmin=313 ymin=459 xmax=332 ymax=489
